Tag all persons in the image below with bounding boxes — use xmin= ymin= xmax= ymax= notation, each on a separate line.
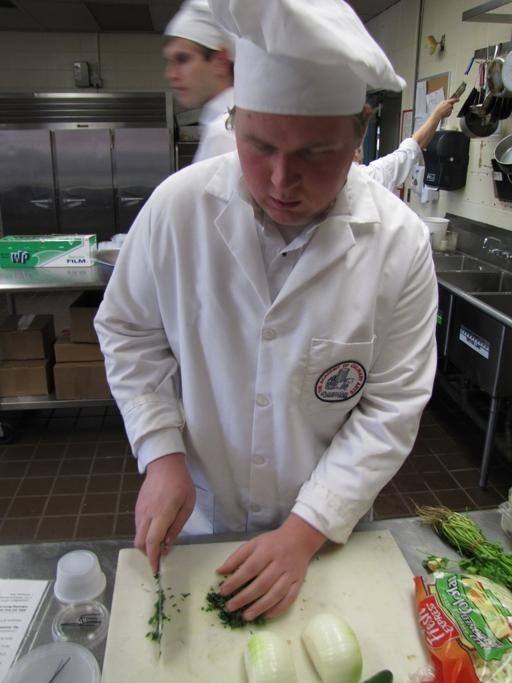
xmin=93 ymin=0 xmax=438 ymax=623
xmin=162 ymin=0 xmax=239 ymax=167
xmin=354 ymin=98 xmax=461 ymax=194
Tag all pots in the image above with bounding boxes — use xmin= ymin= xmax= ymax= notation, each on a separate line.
xmin=459 ymin=63 xmax=498 ymax=138
xmin=495 ymin=134 xmax=512 ymax=183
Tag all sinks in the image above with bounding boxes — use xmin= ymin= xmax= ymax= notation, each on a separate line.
xmin=456 ymin=293 xmax=512 ymax=326
xmin=436 ymin=271 xmax=512 ymax=293
xmin=431 ymin=255 xmax=503 ymax=271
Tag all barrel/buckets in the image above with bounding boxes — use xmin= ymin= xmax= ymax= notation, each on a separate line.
xmin=423 ymin=217 xmax=450 ymax=251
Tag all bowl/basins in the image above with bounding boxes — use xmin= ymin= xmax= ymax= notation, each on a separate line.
xmin=432 ymin=231 xmax=458 ymax=252
xmin=53 ymin=550 xmax=107 ymax=605
xmin=89 ymin=248 xmax=121 ymax=285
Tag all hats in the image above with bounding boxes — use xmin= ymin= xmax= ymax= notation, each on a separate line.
xmin=164 ymin=1 xmax=235 ymax=59
xmin=209 ymin=0 xmax=406 ymax=116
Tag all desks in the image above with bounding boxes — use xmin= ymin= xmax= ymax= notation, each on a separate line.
xmin=0 ymin=508 xmax=512 ymax=662
xmin=1 ymin=268 xmax=116 ymax=412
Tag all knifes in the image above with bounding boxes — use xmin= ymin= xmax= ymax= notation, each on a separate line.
xmin=154 ymin=540 xmax=164 ymax=636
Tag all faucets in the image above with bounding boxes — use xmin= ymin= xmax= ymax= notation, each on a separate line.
xmin=482 ymin=236 xmax=501 ymax=247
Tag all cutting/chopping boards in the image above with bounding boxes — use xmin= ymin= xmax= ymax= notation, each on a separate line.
xmin=100 ymin=527 xmax=438 ymax=683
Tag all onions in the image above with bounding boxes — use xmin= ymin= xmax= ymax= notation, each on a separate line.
xmin=243 ymin=630 xmax=297 ymax=683
xmin=301 ymin=612 xmax=362 ymax=683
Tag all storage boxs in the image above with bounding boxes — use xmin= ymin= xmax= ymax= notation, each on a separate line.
xmin=0 ymin=358 xmax=50 ymax=397
xmin=55 ymin=328 xmax=105 ymax=362
xmin=0 ymin=314 xmax=53 ymax=360
xmin=53 ymin=362 xmax=112 ymax=400
xmin=69 ymin=291 xmax=100 ymax=343
